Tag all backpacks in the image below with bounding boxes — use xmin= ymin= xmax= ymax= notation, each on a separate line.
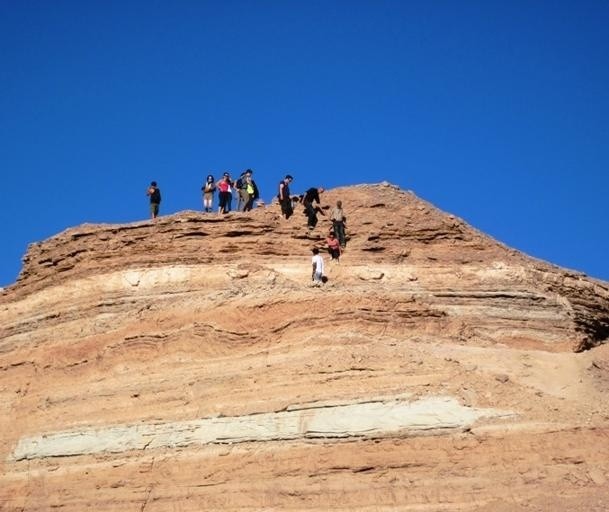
xmin=236 ymin=175 xmax=247 ymax=189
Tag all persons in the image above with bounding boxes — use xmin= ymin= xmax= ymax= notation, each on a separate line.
xmin=332 ymin=199 xmax=347 ymax=246
xmin=201 ymin=168 xmax=259 ymax=213
xmin=277 ymin=175 xmax=294 ymax=219
xmin=312 ymin=248 xmax=324 ymax=283
xmin=147 ymin=181 xmax=162 ymax=219
xmin=303 ymin=201 xmax=326 ymax=230
xmin=299 ymin=187 xmax=325 ymax=209
xmin=323 ymin=230 xmax=342 ymax=264
xmin=329 ymin=222 xmax=348 ymax=237
